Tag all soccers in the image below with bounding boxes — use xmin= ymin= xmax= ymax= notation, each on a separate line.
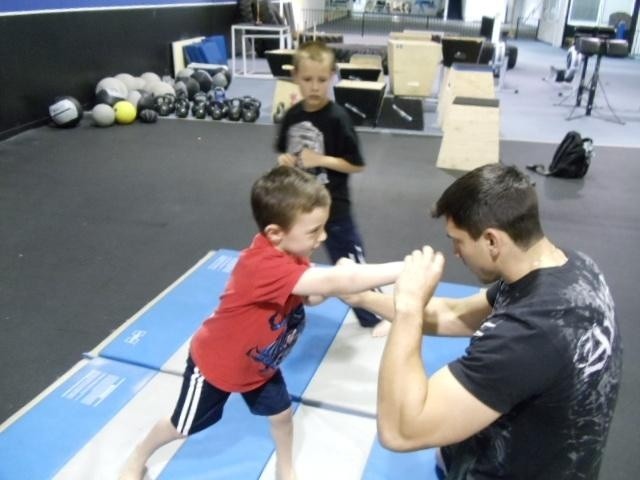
xmin=48 ymin=67 xmax=231 ymax=129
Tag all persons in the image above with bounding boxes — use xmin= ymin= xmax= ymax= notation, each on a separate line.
xmin=121 ymin=165 xmax=405 ymax=480
xmin=332 ymin=159 xmax=624 ymax=480
xmin=271 ymin=39 xmax=391 ymax=328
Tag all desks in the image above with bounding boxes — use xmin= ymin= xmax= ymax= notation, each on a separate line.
xmin=230 ymin=22 xmax=291 ymax=79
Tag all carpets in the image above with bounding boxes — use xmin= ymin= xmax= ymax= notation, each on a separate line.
xmin=1 ymin=249 xmax=489 ymax=480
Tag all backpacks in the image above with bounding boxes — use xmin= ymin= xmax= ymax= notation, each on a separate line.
xmin=526 ymin=129 xmax=593 ymax=178
